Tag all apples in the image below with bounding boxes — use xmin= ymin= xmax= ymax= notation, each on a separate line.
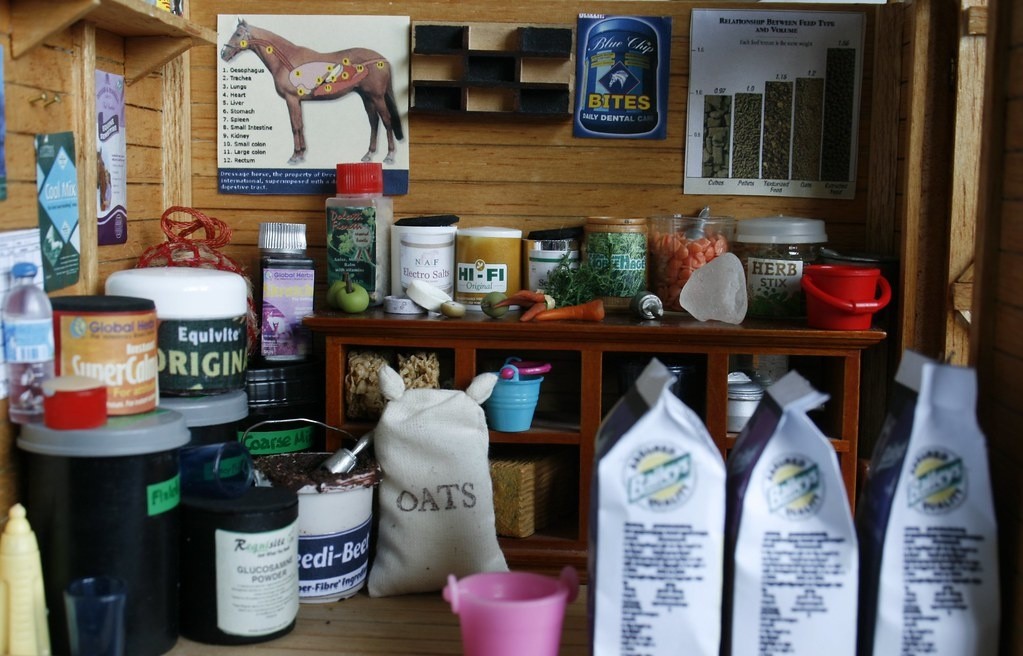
xmin=441 ymin=300 xmax=466 ymax=316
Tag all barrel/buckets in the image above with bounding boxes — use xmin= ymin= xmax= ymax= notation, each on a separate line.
xmin=389 ymin=215 xmax=460 ymax=302
xmin=16 ymin=244 xmax=385 ymax=655
xmin=522 ymin=239 xmax=579 ymax=294
xmin=482 ymin=357 xmax=544 ymax=432
xmin=581 ymin=216 xmax=649 ymax=310
xmin=614 ymin=360 xmax=687 ymax=399
xmin=455 ymin=226 xmax=522 ymax=311
xmin=734 ymin=214 xmax=827 ymax=319
xmin=649 ymin=215 xmax=735 ymax=313
xmin=441 ymin=566 xmax=580 ymax=656
xmin=801 ymin=264 xmax=892 ymax=331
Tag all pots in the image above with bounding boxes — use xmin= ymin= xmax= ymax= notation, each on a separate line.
xmin=728 ymin=371 xmax=764 ymax=433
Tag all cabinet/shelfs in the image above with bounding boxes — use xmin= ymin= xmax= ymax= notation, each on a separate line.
xmin=300 ymin=308 xmax=886 ymax=586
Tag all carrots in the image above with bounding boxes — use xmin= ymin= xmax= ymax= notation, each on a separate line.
xmin=495 ymin=290 xmax=605 ymax=322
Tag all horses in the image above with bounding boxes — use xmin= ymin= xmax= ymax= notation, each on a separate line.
xmin=220 ymin=17 xmax=403 ymax=165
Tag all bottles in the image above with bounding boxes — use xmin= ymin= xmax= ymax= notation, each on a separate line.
xmin=2 ymin=262 xmax=55 ymax=425
xmin=0 ymin=503 xmax=51 ymax=656
xmin=325 ymin=162 xmax=393 ymax=306
xmin=252 ymin=222 xmax=321 ymax=367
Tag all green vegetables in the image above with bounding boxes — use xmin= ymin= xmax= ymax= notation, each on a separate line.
xmin=588 ymin=233 xmax=647 ymax=297
xmin=546 ymin=232 xmax=614 ymax=306
